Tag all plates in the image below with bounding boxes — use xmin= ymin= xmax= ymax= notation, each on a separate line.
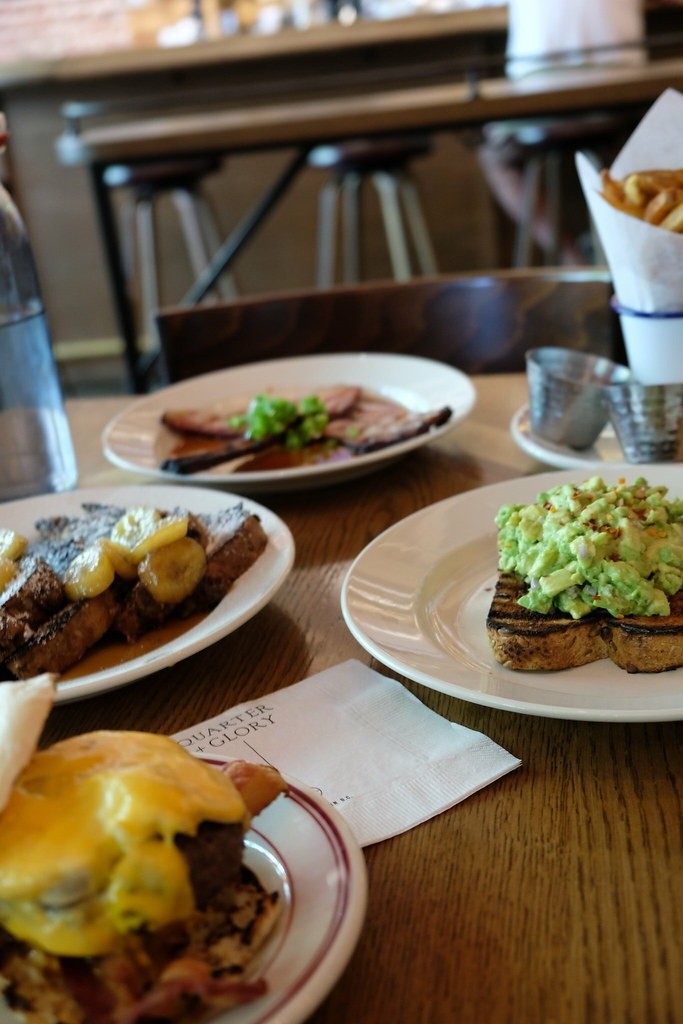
xmin=341 ymin=462 xmax=683 ymax=721
xmin=0 ymin=486 xmax=294 ymax=706
xmin=100 ymin=353 xmax=477 ymax=493
xmin=510 ymin=401 xmax=630 ymax=470
xmin=185 ymin=752 xmax=369 ymax=1024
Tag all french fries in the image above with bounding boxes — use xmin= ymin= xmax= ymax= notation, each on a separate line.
xmin=596 ymin=166 xmax=683 ymax=235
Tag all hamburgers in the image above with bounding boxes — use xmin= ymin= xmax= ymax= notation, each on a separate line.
xmin=0 ymin=668 xmax=297 ymax=1024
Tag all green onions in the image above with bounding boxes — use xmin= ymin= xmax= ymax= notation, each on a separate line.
xmin=233 ymin=394 xmax=328 ymax=449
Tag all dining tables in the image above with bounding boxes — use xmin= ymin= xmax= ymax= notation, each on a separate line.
xmin=0 ymin=371 xmax=683 ymax=1024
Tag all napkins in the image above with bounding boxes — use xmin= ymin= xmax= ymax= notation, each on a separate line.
xmin=170 ymin=658 xmax=523 ymax=848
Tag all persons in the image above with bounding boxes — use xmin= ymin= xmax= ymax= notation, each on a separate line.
xmin=477 ymin=1 xmax=652 ymax=269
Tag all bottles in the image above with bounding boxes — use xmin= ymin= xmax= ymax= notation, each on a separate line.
xmin=0 ymin=184 xmax=79 ymax=503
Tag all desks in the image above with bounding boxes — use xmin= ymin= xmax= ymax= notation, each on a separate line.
xmin=0 ymin=7 xmax=508 ymax=106
xmin=80 ymin=58 xmax=683 ymax=399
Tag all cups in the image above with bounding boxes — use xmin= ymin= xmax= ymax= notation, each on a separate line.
xmin=526 ymin=346 xmax=632 ymax=455
xmin=600 ymin=383 xmax=683 ymax=463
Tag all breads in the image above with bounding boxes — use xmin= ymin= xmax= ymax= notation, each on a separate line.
xmin=487 ymin=570 xmax=683 ymax=674
xmin=0 ymin=503 xmax=268 ymax=678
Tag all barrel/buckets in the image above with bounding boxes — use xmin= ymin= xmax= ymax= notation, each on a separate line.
xmin=610 ymin=297 xmax=683 ymax=384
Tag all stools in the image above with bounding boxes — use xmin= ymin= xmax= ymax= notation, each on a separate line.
xmin=119 ymin=155 xmax=242 ymax=354
xmin=308 ymin=137 xmax=436 ymax=287
xmin=482 ymin=112 xmax=611 ymax=270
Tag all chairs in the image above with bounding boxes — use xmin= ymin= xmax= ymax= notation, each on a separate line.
xmin=154 ymin=266 xmax=629 ymax=374
